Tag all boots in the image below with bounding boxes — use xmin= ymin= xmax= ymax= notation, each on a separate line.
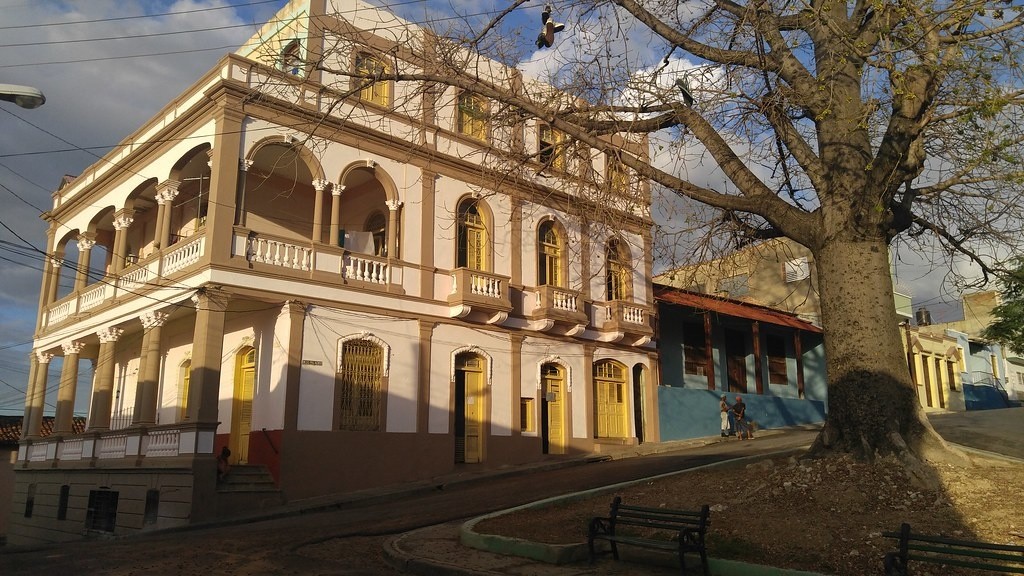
xmin=721 ymin=433 xmax=729 ymax=437
xmin=728 ymin=431 xmax=736 ymax=436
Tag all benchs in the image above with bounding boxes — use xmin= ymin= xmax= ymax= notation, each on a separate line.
xmin=881 ymin=523 xmax=1024 ymax=576
xmin=588 ymin=495 xmax=713 ymax=576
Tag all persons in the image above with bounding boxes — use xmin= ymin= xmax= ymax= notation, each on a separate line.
xmin=720 ymin=394 xmax=736 ymax=437
xmin=217 ymin=446 xmax=231 ymax=480
xmin=732 ymin=396 xmax=755 ymax=440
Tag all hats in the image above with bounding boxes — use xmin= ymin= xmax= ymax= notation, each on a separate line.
xmin=721 ymin=394 xmax=728 ymax=398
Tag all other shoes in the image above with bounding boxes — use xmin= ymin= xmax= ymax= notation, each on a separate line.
xmin=739 ymin=438 xmax=743 ymax=440
xmin=748 ymin=437 xmax=755 ymax=439
